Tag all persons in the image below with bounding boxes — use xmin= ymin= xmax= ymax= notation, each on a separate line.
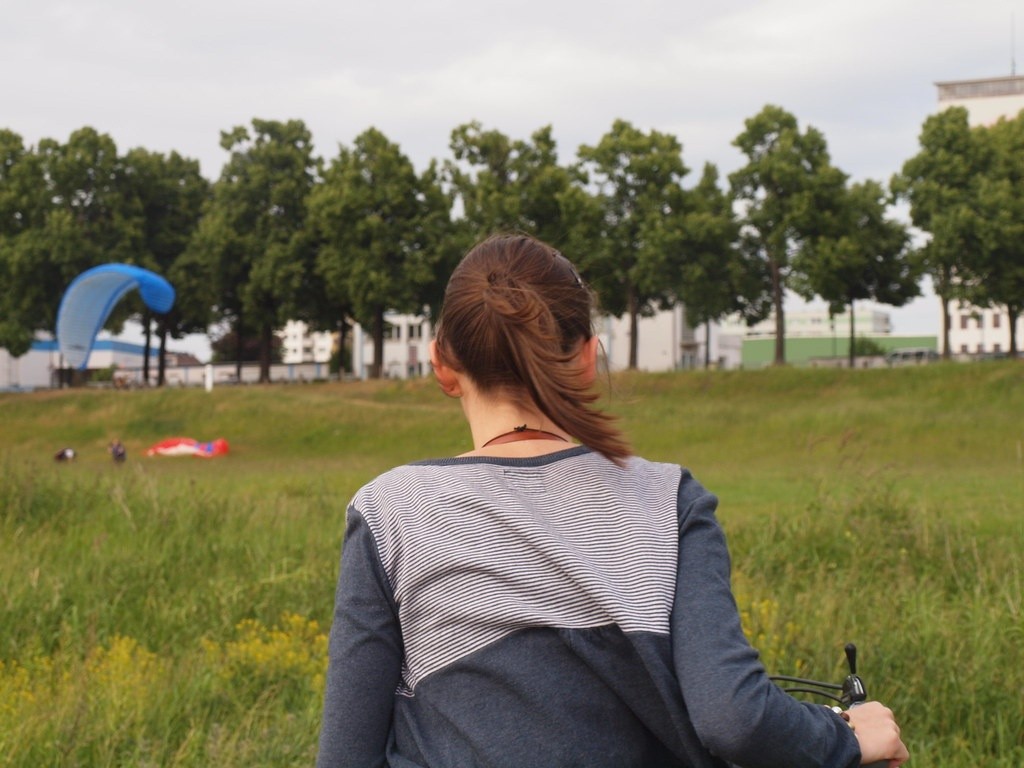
xmin=314 ymin=234 xmax=909 ymax=768
xmin=108 ymin=437 xmax=125 ymax=463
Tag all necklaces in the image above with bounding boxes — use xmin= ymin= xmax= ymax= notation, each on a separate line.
xmin=483 ymin=426 xmax=568 ymax=448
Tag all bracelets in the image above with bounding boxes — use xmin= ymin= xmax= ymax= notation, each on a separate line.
xmin=824 ymin=705 xmax=858 ymax=738
